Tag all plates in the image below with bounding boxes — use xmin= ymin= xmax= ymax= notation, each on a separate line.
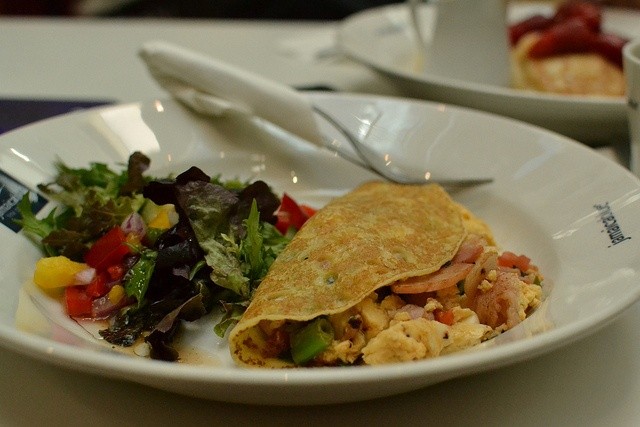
xmin=0 ymin=90 xmax=638 ymax=409
xmin=2 ymin=17 xmax=406 ymax=104
xmin=336 ymin=1 xmax=639 ymax=140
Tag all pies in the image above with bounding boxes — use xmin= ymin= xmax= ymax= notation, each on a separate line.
xmin=228 ymin=182 xmax=542 ymax=369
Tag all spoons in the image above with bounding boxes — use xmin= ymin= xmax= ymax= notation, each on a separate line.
xmin=139 ymin=39 xmax=493 ymax=193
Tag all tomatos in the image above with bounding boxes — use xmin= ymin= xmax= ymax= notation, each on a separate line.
xmin=65 ymin=227 xmax=135 ymax=315
